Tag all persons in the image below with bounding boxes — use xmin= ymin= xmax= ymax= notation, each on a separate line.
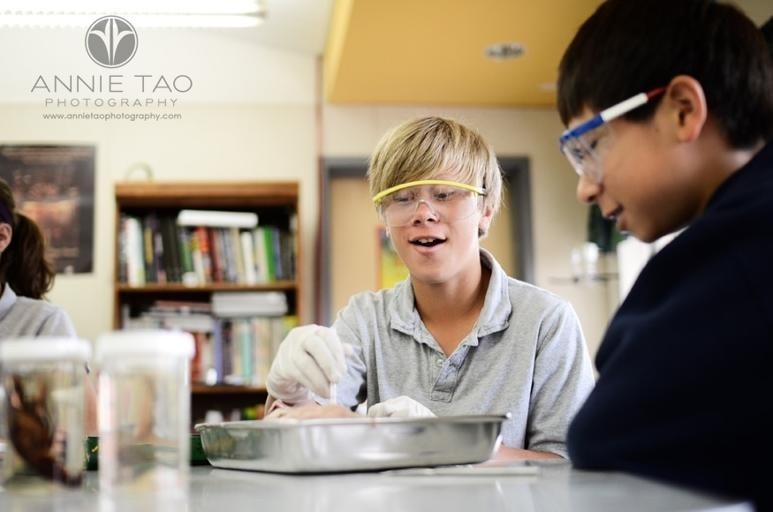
xmin=555 ymin=2 xmax=773 ymax=511
xmin=263 ymin=115 xmax=596 ymax=463
xmin=1 ymin=177 xmax=159 ymax=441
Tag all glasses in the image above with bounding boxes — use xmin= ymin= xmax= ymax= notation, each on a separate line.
xmin=372 ymin=180 xmax=487 ymax=226
xmin=560 ymin=88 xmax=667 ymax=184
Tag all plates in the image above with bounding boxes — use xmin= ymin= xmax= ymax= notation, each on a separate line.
xmin=193 ymin=410 xmax=514 ymax=475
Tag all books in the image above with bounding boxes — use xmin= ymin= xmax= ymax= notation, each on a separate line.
xmin=118 ymin=208 xmax=295 ymax=423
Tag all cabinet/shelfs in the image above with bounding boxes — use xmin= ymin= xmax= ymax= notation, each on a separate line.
xmin=113 ymin=181 xmax=302 ymax=421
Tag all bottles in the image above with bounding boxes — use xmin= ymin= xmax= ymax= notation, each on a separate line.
xmin=0 ymin=335 xmax=90 ymax=500
xmin=93 ymin=326 xmax=197 ymax=500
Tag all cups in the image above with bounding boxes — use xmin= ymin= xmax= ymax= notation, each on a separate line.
xmin=570 ymin=241 xmax=600 ymax=280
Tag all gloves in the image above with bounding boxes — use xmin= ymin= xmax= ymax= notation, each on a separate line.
xmin=265 ymin=324 xmax=347 ymax=401
xmin=368 ymin=396 xmax=436 ymax=417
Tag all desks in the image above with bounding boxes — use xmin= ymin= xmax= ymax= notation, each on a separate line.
xmin=0 ymin=462 xmax=754 ymax=512
